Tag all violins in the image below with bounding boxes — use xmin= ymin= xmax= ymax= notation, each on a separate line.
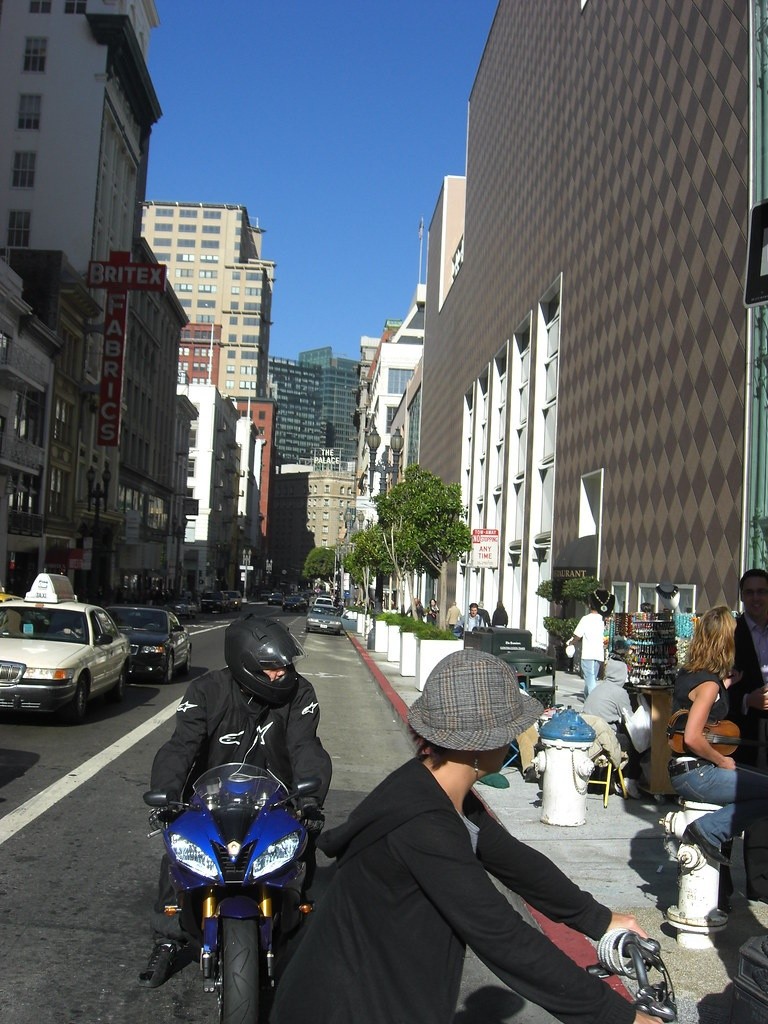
xmin=666 ymin=709 xmax=768 ymax=757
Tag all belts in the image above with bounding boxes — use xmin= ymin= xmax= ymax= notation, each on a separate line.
xmin=667 ymin=758 xmax=713 ymax=777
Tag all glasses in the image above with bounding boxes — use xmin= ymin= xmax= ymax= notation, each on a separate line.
xmin=741 ymin=588 xmax=768 ymax=598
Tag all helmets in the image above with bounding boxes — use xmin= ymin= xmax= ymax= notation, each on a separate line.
xmin=224 ymin=612 xmax=307 ymax=703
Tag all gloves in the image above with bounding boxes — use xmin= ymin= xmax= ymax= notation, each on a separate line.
xmin=157 ymin=790 xmax=188 ymax=824
xmin=298 ymin=797 xmax=325 ymax=833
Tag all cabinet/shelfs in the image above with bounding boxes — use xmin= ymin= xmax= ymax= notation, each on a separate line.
xmin=625 ymin=686 xmax=678 ymax=804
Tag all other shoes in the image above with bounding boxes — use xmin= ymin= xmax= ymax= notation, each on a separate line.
xmin=617 ymin=777 xmax=641 ymax=799
xmin=683 ymin=822 xmax=734 ymax=866
xmin=610 ymin=781 xmax=623 ymax=795
xmin=747 ymin=895 xmax=768 ymax=904
xmin=718 ymin=895 xmax=733 ymax=914
xmin=525 ymin=767 xmax=539 ymax=784
xmin=138 ymin=944 xmax=177 ymax=988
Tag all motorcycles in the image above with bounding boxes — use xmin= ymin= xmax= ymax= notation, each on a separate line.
xmin=142 ymin=762 xmax=322 ymax=1023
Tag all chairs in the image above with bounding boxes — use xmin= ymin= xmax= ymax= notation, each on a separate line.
xmin=115 ymin=613 xmax=130 ymax=627
xmin=580 ymin=715 xmax=628 ymax=808
xmin=19 ymin=612 xmax=37 ymax=635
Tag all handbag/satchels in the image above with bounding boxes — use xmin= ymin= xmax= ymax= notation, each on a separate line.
xmin=622 ymin=693 xmax=652 ymax=754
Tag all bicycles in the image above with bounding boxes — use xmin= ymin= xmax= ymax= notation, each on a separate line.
xmin=587 ymin=929 xmax=677 ymax=1023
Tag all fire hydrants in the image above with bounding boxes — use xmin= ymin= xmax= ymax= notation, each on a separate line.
xmin=530 ymin=708 xmax=596 ymax=827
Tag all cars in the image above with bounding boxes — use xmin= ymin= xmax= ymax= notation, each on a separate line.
xmin=267 ymin=591 xmax=309 ymax=612
xmin=164 ymin=601 xmax=198 ymax=620
xmin=105 ymin=604 xmax=193 ymax=683
xmin=0 ymin=573 xmax=132 ymax=725
xmin=305 ymin=595 xmax=343 ymax=635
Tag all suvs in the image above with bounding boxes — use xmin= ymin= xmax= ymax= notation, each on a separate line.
xmin=201 ymin=590 xmax=242 ymax=613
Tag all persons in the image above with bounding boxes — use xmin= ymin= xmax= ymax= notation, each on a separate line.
xmin=64 ymin=617 xmax=83 ymax=639
xmin=492 ymin=601 xmax=508 ymax=628
xmin=478 ymin=601 xmax=492 ymax=627
xmin=0 ymin=609 xmax=9 ymax=630
xmin=426 ymin=598 xmax=440 ymax=625
xmin=265 ymin=648 xmax=665 ymax=1024
xmin=718 ymin=569 xmax=768 ymax=914
xmin=451 ymin=603 xmax=485 ymax=639
xmin=138 ymin=610 xmax=333 ymax=988
xmin=579 ymin=659 xmax=641 ymax=800
xmin=6 ymin=578 xmax=161 ymax=606
xmin=152 ymin=612 xmax=165 ymax=629
xmin=446 ymin=601 xmax=462 ymax=629
xmin=565 ymin=593 xmax=604 ymax=703
xmin=405 ymin=597 xmax=426 ymax=622
xmin=641 ymin=602 xmax=652 ymax=612
xmin=667 ymin=605 xmax=768 ymax=865
xmin=314 ymin=587 xmax=321 ymax=597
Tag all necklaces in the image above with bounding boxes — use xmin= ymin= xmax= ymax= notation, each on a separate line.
xmin=603 ymin=611 xmax=633 ymax=665
xmin=673 ymin=611 xmax=695 ymax=638
xmin=594 ymin=589 xmax=610 ymax=612
xmin=656 ymin=585 xmax=679 ymax=599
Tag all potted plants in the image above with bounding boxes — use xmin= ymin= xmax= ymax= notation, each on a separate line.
xmin=536 ymin=575 xmax=576 ymax=671
xmin=344 ymin=605 xmax=466 ymax=692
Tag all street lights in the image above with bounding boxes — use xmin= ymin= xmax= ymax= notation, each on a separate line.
xmin=86 ymin=462 xmax=111 ymax=602
xmin=366 ymin=427 xmax=405 ymax=649
xmin=325 ymin=548 xmax=337 ymax=594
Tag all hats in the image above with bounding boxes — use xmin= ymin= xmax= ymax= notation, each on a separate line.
xmin=408 ymin=652 xmax=544 ymax=752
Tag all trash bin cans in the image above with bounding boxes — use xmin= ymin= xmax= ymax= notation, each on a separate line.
xmin=464 ymin=627 xmax=532 ymax=656
xmin=498 ymin=650 xmax=555 ymax=710
